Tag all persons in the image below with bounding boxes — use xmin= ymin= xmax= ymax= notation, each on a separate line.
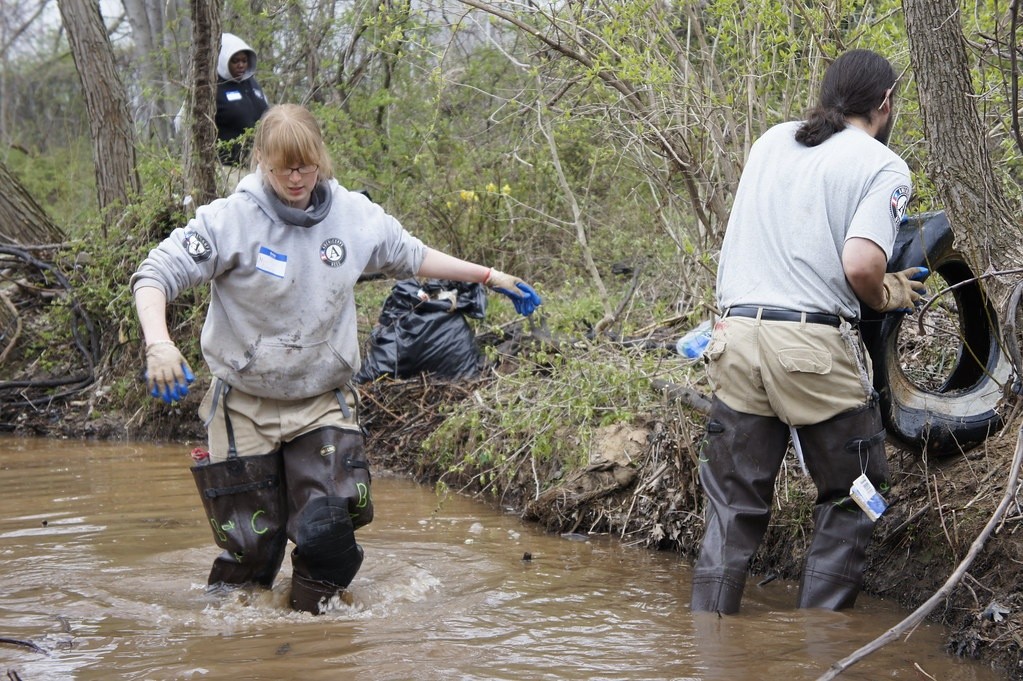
xmin=174 ymin=33 xmax=270 ymax=195
xmin=129 ymin=102 xmax=541 ymax=614
xmin=690 ymin=49 xmax=929 ymax=613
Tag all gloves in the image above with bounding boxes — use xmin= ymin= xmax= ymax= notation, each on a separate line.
xmin=870 ymin=266 xmax=929 ymax=315
xmin=484 ymin=266 xmax=541 ymax=317
xmin=145 ymin=340 xmax=195 ymax=404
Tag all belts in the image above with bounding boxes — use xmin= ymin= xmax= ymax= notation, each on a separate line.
xmin=721 ymin=306 xmax=854 ymax=328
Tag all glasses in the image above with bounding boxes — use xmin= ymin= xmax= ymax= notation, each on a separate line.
xmin=264 ymin=158 xmax=320 ymax=177
